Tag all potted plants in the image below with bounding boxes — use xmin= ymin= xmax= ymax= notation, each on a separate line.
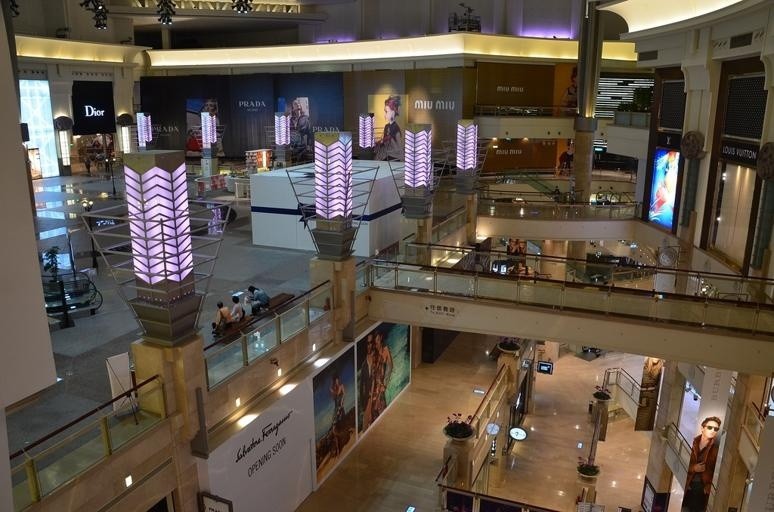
xmin=224 ymin=167 xmax=243 ymax=192
xmin=39 ymin=245 xmax=61 ymax=292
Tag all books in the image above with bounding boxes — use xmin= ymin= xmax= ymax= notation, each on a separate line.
xmin=577 ymin=502 xmax=592 ymax=512
xmin=617 ymin=507 xmax=632 ymax=512
xmin=591 ymin=504 xmax=606 ymax=512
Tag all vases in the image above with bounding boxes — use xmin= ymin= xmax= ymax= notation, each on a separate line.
xmin=443 ymin=428 xmax=476 ymax=443
xmin=576 ymin=467 xmax=600 ymax=482
xmin=593 ymin=395 xmax=612 ymax=403
xmin=497 ymin=343 xmax=520 ymax=354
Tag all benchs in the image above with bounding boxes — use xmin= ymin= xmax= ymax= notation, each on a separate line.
xmin=213 ymin=315 xmax=254 ymax=339
xmin=262 ymin=293 xmax=295 ymax=316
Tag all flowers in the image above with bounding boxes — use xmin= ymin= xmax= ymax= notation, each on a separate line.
xmin=577 ymin=455 xmax=599 ymax=475
xmin=594 ymin=384 xmax=611 ymax=399
xmin=497 ymin=337 xmax=520 ymax=350
xmin=446 ymin=412 xmax=475 ymax=436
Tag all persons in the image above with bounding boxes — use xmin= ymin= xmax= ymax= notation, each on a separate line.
xmin=358 ymin=334 xmax=386 ymax=435
xmin=296 ymin=144 xmax=309 ymax=165
xmin=370 ymin=331 xmax=393 ymax=417
xmin=209 ymin=301 xmax=230 ymax=338
xmin=245 ymin=285 xmax=272 ymax=317
xmin=557 ymin=138 xmax=575 ymax=177
xmin=200 ymin=97 xmax=219 ymax=115
xmin=682 ymin=416 xmax=722 ymax=512
xmin=83 ymin=151 xmax=117 ymax=174
xmin=517 ymin=239 xmax=527 ymax=255
xmin=507 ymin=239 xmax=520 ymax=255
xmin=105 ymin=133 xmax=114 ymax=153
xmin=553 ymin=185 xmax=561 ymax=204
xmin=634 ymin=355 xmax=666 ymax=431
xmin=290 ymin=143 xmax=298 ymax=165
xmin=228 ymin=295 xmax=242 ymax=323
xmin=288 ymin=96 xmax=310 ymax=152
xmin=327 ymin=376 xmax=354 ymax=460
xmin=372 ymin=96 xmax=403 ymax=161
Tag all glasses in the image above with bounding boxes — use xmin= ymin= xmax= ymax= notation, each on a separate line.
xmin=704 ymin=424 xmax=720 ymax=431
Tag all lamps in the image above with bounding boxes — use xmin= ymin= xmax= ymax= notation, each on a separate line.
xmin=402 ymin=123 xmax=432 ymax=218
xmin=157 ymin=0 xmax=176 ymax=25
xmin=455 ymin=118 xmax=477 ymax=193
xmin=138 ymin=113 xmax=153 ymax=151
xmin=117 ymin=114 xmax=133 ymax=154
xmin=79 ymin=0 xmax=109 ymax=29
xmin=230 ymin=0 xmax=253 ymax=13
xmin=275 ymin=112 xmax=293 ymax=161
xmin=357 ymin=113 xmax=376 ymax=160
xmin=54 ymin=115 xmax=72 ymax=167
xmin=10 ymin=0 xmax=20 ymax=19
xmin=123 ymin=150 xmax=203 ymax=346
xmin=314 ymin=131 xmax=356 ymax=261
xmin=202 ymin=112 xmax=218 ymax=158
